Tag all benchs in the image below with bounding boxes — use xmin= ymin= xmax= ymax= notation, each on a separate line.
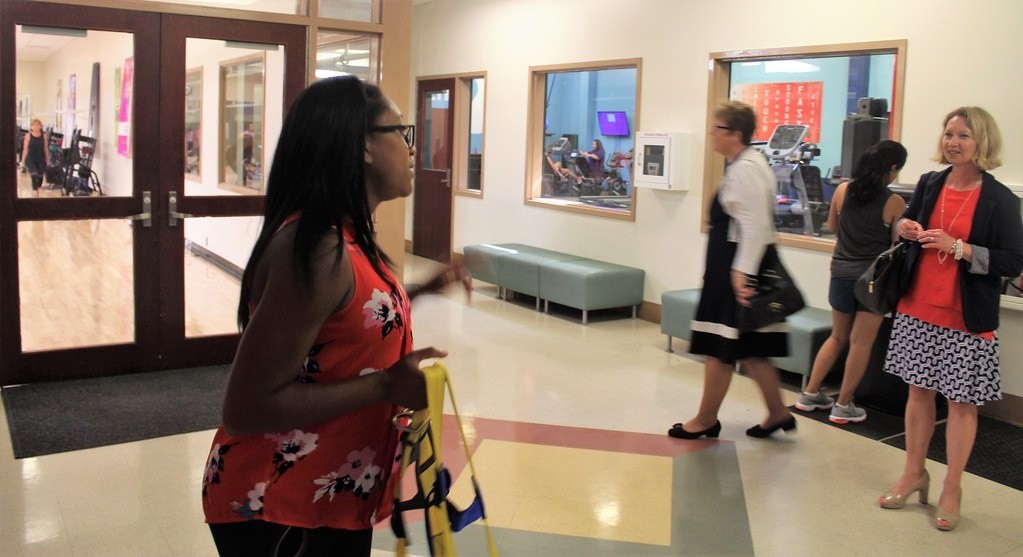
xmin=463 ymin=244 xmax=646 ymax=326
xmin=661 ymin=288 xmax=838 ymax=395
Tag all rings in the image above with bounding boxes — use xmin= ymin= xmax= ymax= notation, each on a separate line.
xmin=931 ymin=237 xmax=935 ymax=242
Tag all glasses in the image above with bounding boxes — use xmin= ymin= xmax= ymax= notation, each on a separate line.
xmin=711 ymin=125 xmax=734 ymax=132
xmin=369 ymin=124 xmax=415 ymax=149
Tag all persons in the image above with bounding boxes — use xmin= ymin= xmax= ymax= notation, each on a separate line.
xmin=186 ymin=127 xmax=200 ymax=171
xmin=202 ymin=75 xmax=472 ymax=557
xmin=668 ymin=102 xmax=797 ymax=438
xmin=880 ymin=107 xmax=1023 ymax=530
xmin=20 ymin=120 xmax=49 ymax=197
xmin=795 ymin=139 xmax=907 ymax=422
xmin=240 ymin=122 xmax=253 ymax=165
xmin=580 ymin=140 xmax=606 ymax=177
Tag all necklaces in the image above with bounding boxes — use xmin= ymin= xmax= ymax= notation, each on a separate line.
xmin=938 ymin=173 xmax=984 ymax=264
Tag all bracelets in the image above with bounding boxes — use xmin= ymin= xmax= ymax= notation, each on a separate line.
xmin=950 ymin=239 xmax=963 ymax=259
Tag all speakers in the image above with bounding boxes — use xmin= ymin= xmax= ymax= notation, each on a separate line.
xmin=839 ymin=116 xmax=891 ymax=178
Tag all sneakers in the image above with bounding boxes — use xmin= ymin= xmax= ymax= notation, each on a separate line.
xmin=795 ymin=392 xmax=834 ymax=412
xmin=828 ymin=402 xmax=867 ymax=424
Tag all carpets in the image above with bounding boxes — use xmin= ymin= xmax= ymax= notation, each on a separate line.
xmin=0 ymin=364 xmax=232 ymax=460
xmin=787 ymin=403 xmax=1023 ymax=492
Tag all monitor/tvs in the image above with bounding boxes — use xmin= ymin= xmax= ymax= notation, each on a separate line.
xmin=765 ymin=124 xmax=809 ymax=158
xmin=597 ymin=111 xmax=629 ymax=137
xmin=552 ymin=137 xmax=568 ymax=151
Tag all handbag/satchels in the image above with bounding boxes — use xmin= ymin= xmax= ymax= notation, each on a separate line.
xmin=734 ymin=243 xmax=805 ymax=333
xmin=853 ymin=237 xmax=920 ymax=315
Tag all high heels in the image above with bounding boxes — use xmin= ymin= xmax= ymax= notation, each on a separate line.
xmin=746 ymin=417 xmax=796 ymax=438
xmin=669 ymin=420 xmax=721 ymax=439
xmin=879 ymin=469 xmax=930 ymax=509
xmin=935 ymin=487 xmax=962 ymax=531
